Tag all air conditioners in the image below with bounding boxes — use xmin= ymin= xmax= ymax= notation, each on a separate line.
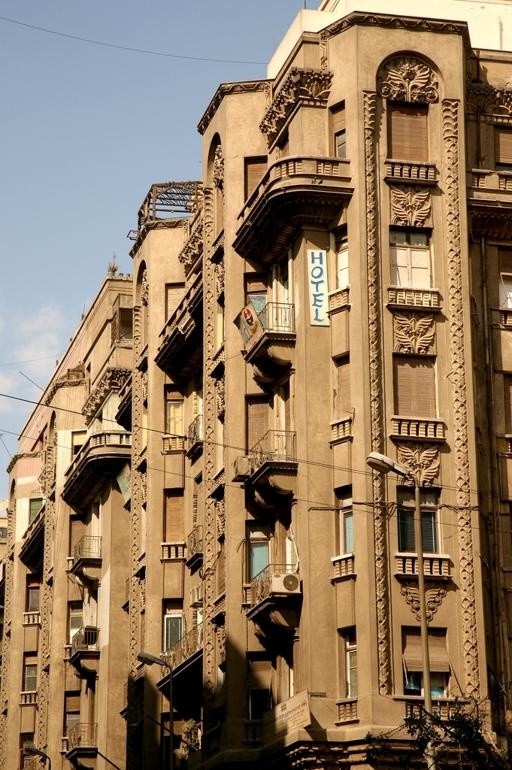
xmin=271 ymin=572 xmax=302 ymax=594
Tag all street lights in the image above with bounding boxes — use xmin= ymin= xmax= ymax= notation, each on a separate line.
xmin=365 ymin=452 xmax=437 ymax=768
xmin=135 ymin=650 xmax=175 ymax=768
xmin=24 ymin=744 xmax=53 ymax=770
